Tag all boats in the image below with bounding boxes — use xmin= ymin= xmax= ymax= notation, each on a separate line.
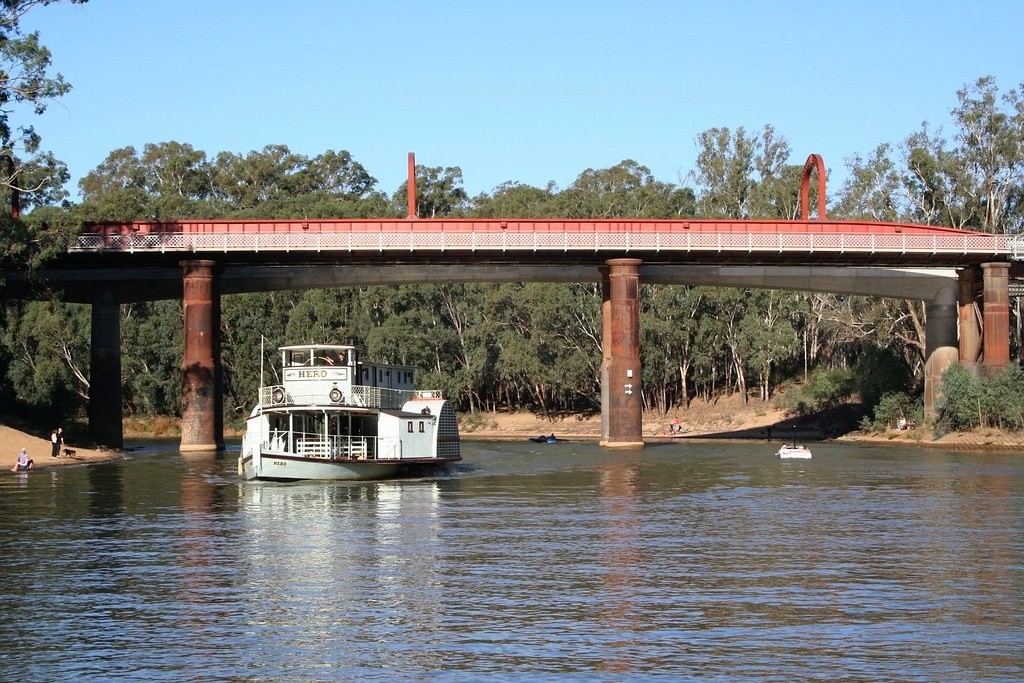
xmin=237 ymin=333 xmax=463 ymax=481
xmin=780 ymin=424 xmax=813 ymax=459
xmin=528 ymin=436 xmax=557 ymax=444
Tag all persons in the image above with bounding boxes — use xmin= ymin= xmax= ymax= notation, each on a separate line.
xmin=337 ymin=353 xmax=346 ymax=366
xmin=896 ymin=415 xmax=906 ymax=430
xmin=52 ymin=428 xmax=64 ymax=459
xmin=17 ymin=448 xmax=28 ymax=464
xmin=674 ymin=417 xmax=682 ymax=432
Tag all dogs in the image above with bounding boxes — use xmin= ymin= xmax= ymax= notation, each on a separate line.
xmin=62 ymin=449 xmax=77 ymax=458
